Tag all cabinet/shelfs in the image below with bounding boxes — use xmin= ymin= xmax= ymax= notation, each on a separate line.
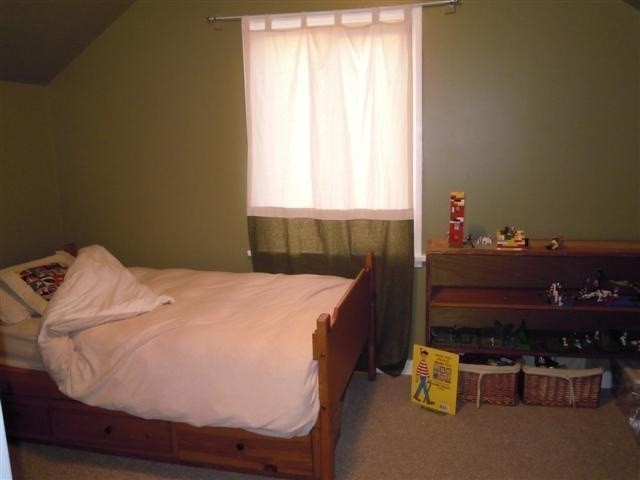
xmin=0 ymin=394 xmax=318 ymax=480
xmin=423 ymin=236 xmax=640 ymax=360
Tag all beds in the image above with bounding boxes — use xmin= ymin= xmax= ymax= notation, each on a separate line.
xmin=0 ymin=248 xmax=377 ymax=480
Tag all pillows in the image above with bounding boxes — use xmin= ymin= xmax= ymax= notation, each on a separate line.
xmin=0 ymin=250 xmax=78 ymax=326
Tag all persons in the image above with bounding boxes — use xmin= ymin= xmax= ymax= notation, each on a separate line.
xmin=444 ymin=192 xmax=640 ymax=371
xmin=412 ymin=349 xmax=435 ymax=405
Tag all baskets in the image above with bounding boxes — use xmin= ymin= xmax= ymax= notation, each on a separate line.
xmin=520 ymin=361 xmax=604 ymax=409
xmin=457 ymin=357 xmax=522 ymax=407
